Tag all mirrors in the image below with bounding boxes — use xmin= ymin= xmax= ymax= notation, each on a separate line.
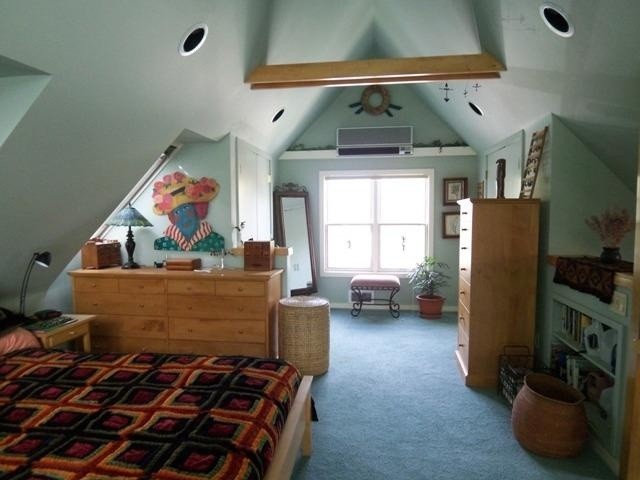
xmin=273 ymin=182 xmax=319 ymax=300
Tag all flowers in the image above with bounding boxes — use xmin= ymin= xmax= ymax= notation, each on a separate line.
xmin=585 ymin=209 xmax=635 ymax=248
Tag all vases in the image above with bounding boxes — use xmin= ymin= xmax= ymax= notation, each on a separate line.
xmin=601 ymin=245 xmax=622 ymax=264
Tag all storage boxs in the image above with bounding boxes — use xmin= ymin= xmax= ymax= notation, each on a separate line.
xmin=243 ymin=240 xmax=276 ymax=272
xmin=80 ymin=237 xmax=123 ymax=269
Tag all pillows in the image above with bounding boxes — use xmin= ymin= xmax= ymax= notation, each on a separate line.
xmin=1 ymin=307 xmax=43 ymax=355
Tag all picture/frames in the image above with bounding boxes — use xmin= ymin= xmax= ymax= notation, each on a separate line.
xmin=442 ymin=211 xmax=460 ymax=239
xmin=443 ymin=176 xmax=469 ymax=206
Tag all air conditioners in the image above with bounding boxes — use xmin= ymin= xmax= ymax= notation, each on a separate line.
xmin=336 ymin=125 xmax=415 ymax=158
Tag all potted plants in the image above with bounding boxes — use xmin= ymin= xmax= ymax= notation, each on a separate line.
xmin=405 ymin=256 xmax=453 ymax=320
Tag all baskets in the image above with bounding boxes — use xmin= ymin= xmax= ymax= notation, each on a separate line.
xmin=496 ymin=345 xmax=554 ymax=409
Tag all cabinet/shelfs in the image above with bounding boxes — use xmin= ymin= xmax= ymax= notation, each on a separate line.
xmin=166 ymin=267 xmax=285 ymax=365
xmin=548 ymin=256 xmax=640 ymax=478
xmin=455 ymin=198 xmax=541 ymax=390
xmin=65 ymin=266 xmax=166 ymax=355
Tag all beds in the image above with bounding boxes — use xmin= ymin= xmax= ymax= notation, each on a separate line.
xmin=0 ymin=346 xmax=319 ymax=479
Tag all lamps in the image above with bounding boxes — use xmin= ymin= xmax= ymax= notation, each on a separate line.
xmin=104 ymin=201 xmax=154 ymax=269
xmin=16 ymin=249 xmax=54 ymax=325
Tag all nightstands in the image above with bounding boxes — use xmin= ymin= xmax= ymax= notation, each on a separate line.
xmin=29 ymin=313 xmax=98 ymax=354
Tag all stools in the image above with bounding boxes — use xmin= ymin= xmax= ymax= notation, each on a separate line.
xmin=276 ymin=296 xmax=330 ymax=378
xmin=350 ymin=274 xmax=402 ymax=319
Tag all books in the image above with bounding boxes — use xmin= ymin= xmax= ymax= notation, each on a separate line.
xmin=554 ymin=302 xmax=602 ymax=403
xmin=25 ymin=313 xmax=78 ymax=331
